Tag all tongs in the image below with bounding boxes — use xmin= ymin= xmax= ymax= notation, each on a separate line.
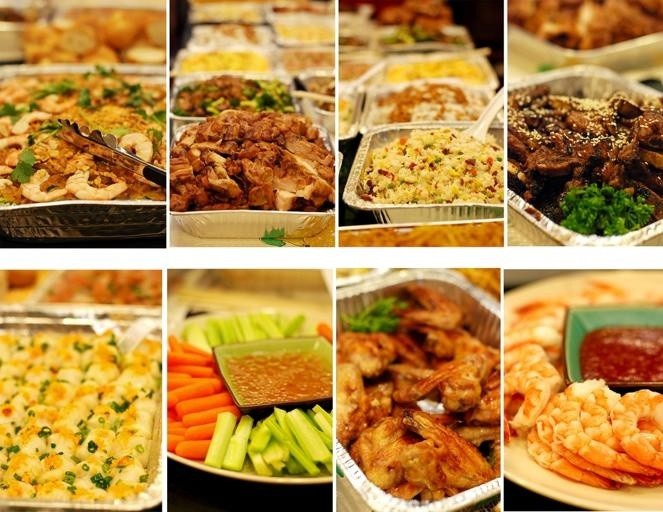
xmin=53 ymin=115 xmax=167 ymax=195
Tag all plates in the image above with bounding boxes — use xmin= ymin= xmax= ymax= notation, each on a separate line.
xmin=504 ymin=271 xmax=662 ymax=511
xmin=167 ymin=307 xmax=333 ymax=485
